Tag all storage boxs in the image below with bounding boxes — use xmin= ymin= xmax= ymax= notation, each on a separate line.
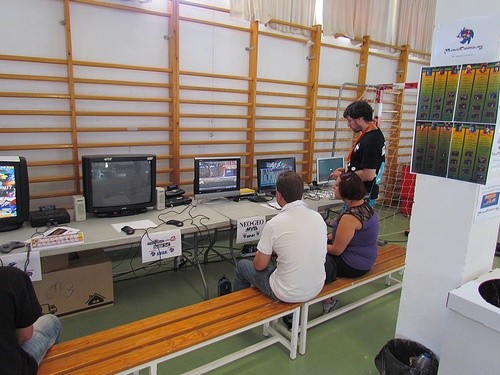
xmin=32 ymin=248 xmax=113 ymax=317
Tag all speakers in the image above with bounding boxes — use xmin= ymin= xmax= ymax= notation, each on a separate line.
xmin=154 ymin=187 xmax=165 ymax=211
xmin=73 ymin=195 xmax=86 ymax=221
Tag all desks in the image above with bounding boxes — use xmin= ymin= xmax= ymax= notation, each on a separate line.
xmin=0 ymin=197 xmax=230 ymax=301
xmin=199 ymin=178 xmax=344 ymax=272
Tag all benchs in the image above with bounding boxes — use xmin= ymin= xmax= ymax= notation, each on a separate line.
xmin=34 ymin=286 xmax=300 ymax=375
xmin=272 ymin=245 xmax=406 ymax=356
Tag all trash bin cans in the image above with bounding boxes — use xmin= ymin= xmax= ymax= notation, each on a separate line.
xmin=401 ymin=165 xmax=416 ymax=216
xmin=381 ymin=339 xmax=439 ymax=375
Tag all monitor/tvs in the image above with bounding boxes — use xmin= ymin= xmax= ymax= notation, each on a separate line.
xmin=316 ymin=156 xmax=344 ymax=184
xmin=0 ymin=157 xmax=30 ymax=232
xmin=256 ymin=156 xmax=296 ymax=193
xmin=194 ymin=156 xmax=240 ymax=205
xmin=81 ymin=153 xmax=156 ymax=217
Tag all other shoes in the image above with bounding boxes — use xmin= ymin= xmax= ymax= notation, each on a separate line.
xmin=283 ymin=313 xmax=292 ymax=329
xmin=321 ymin=299 xmax=339 ymax=316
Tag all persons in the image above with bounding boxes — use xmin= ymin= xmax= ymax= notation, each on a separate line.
xmin=320 ymin=170 xmax=379 ymax=316
xmin=0 ymin=266 xmax=61 ymax=375
xmin=329 ymin=101 xmax=386 ymax=212
xmin=233 ymin=171 xmax=327 ymax=330
